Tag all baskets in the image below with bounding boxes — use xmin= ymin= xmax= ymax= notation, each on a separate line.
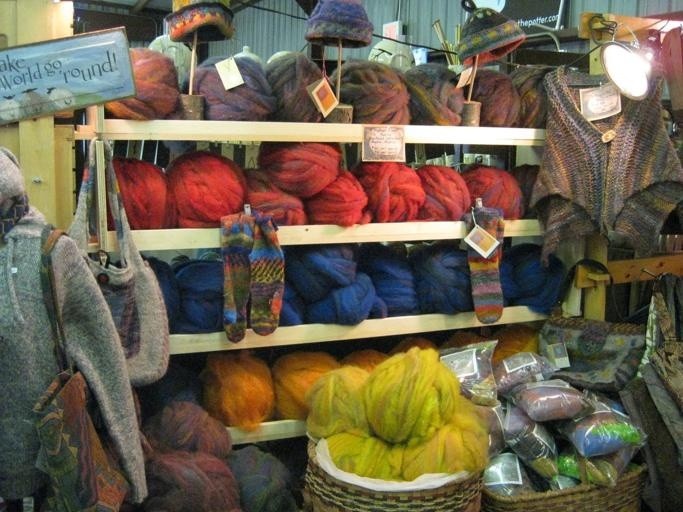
xmin=483 ymin=457 xmax=649 ymax=512
xmin=302 ymin=436 xmax=491 ymax=512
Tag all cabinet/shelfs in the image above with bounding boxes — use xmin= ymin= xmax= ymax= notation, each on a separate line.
xmin=79 ymin=116 xmax=591 ymax=447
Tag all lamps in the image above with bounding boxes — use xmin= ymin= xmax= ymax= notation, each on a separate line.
xmin=600 ymin=40 xmax=655 ymax=102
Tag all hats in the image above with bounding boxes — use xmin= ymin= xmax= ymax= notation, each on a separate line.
xmin=304 ymin=0 xmax=373 ymax=48
xmin=456 ymin=0 xmax=526 ymax=65
xmin=165 ymin=0 xmax=235 ymax=41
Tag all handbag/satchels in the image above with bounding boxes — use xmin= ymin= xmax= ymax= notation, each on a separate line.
xmin=649 ymin=272 xmax=683 ymax=408
xmin=536 ymin=258 xmax=647 ymax=396
xmin=67 ymin=133 xmax=170 ymax=389
xmin=31 ymin=224 xmax=132 ymax=512
